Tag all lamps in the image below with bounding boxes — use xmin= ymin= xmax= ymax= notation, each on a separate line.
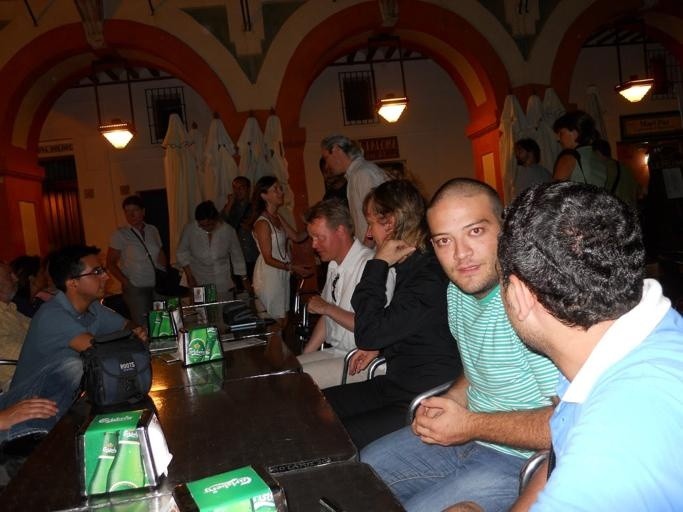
xmin=610 ymin=16 xmax=656 ymax=105
xmin=366 ymin=33 xmax=410 ymax=124
xmin=89 ymin=51 xmax=138 ymax=151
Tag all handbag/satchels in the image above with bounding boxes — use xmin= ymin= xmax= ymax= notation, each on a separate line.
xmin=79 ymin=328 xmax=153 ymax=408
xmin=155 ymin=268 xmax=188 ymax=296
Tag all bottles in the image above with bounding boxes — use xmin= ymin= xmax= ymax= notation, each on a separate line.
xmin=87 ymin=430 xmax=118 ymax=494
xmin=151 ymin=312 xmax=222 ymax=364
xmin=105 ymin=429 xmax=144 ymax=491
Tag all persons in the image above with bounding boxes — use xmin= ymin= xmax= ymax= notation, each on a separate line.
xmin=0 ymin=347 xmax=82 ymax=487
xmin=3 ymin=134 xmax=390 ymax=391
xmin=506 ymin=111 xmax=641 ymax=212
xmin=320 ymin=180 xmax=462 ymax=454
xmin=439 ymin=179 xmax=683 ymax=511
xmin=358 ymin=178 xmax=561 ymax=512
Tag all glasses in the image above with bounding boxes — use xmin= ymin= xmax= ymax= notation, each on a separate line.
xmin=74 ymin=264 xmax=106 ymax=279
xmin=332 ymin=276 xmax=339 ymax=302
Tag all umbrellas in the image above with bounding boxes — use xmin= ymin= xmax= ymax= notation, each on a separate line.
xmin=499 ymin=86 xmax=608 ymax=206
xmin=161 ymin=107 xmax=295 ymax=275
